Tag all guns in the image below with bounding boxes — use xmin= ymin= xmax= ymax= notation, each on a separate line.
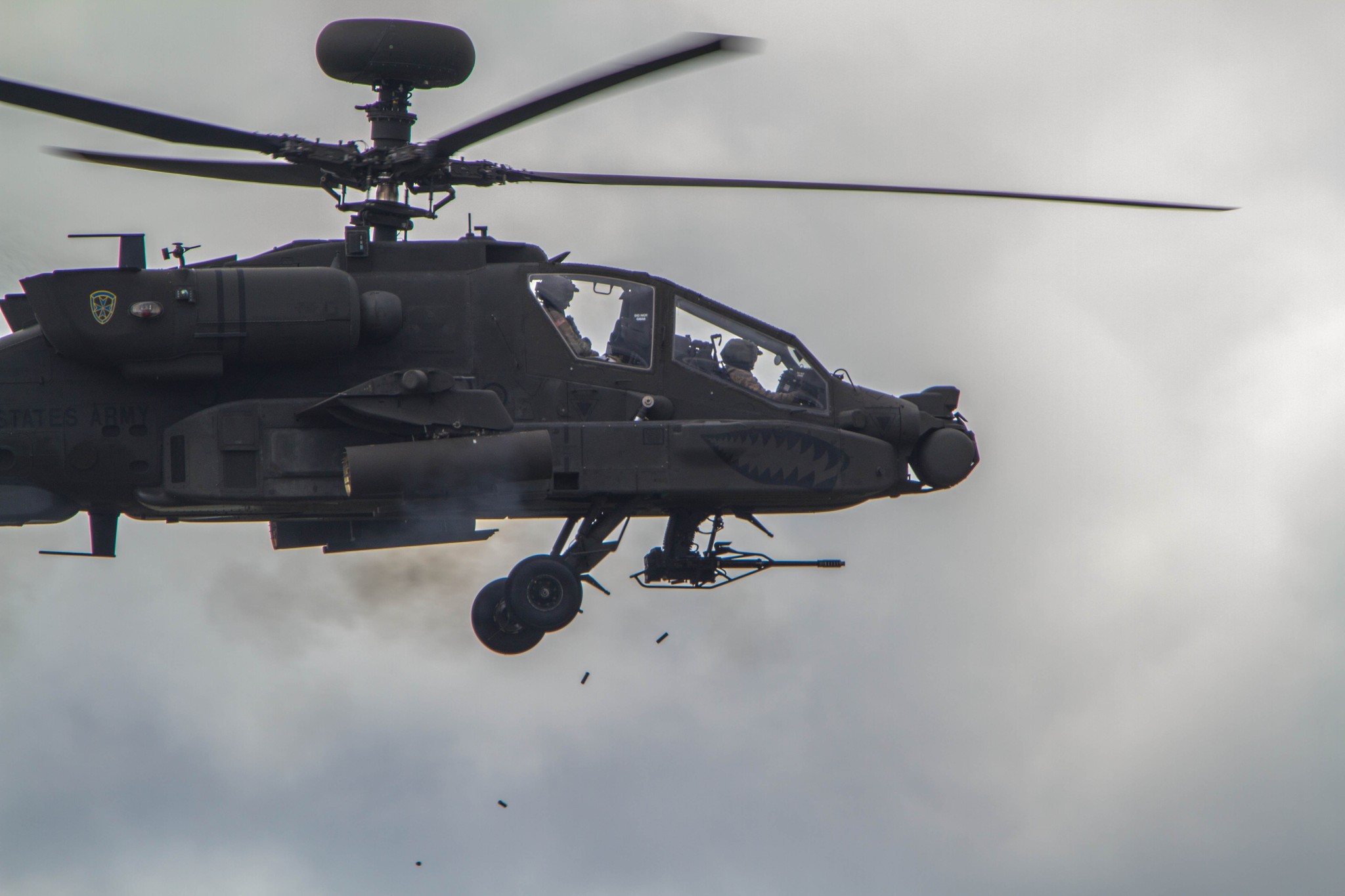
xmin=644 ymin=546 xmax=846 ymax=588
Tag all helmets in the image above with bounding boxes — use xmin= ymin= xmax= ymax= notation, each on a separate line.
xmin=720 ymin=338 xmax=762 ymax=371
xmin=535 ymin=276 xmax=579 ymax=313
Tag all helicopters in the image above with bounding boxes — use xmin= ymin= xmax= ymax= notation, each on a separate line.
xmin=0 ymin=17 xmax=1242 ymax=656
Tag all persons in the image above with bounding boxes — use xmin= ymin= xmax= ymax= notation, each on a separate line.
xmin=536 ymin=276 xmax=610 ymax=361
xmin=721 ymin=338 xmax=804 ymax=404
